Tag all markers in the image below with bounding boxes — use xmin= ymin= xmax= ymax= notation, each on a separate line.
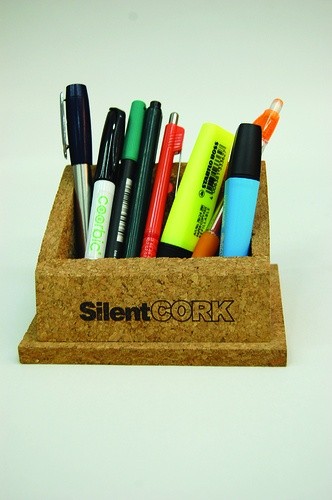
xmin=219 ymin=124 xmax=262 ymax=257
xmin=158 ymin=122 xmax=235 ymax=258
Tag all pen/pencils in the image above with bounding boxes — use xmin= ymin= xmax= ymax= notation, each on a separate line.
xmin=188 ymin=98 xmax=283 ymax=258
xmin=139 ymin=111 xmax=184 ymax=258
xmin=106 ymin=100 xmax=162 ymax=259
xmin=85 ymin=106 xmax=125 ymax=259
xmin=60 ymin=84 xmax=91 ymax=247
